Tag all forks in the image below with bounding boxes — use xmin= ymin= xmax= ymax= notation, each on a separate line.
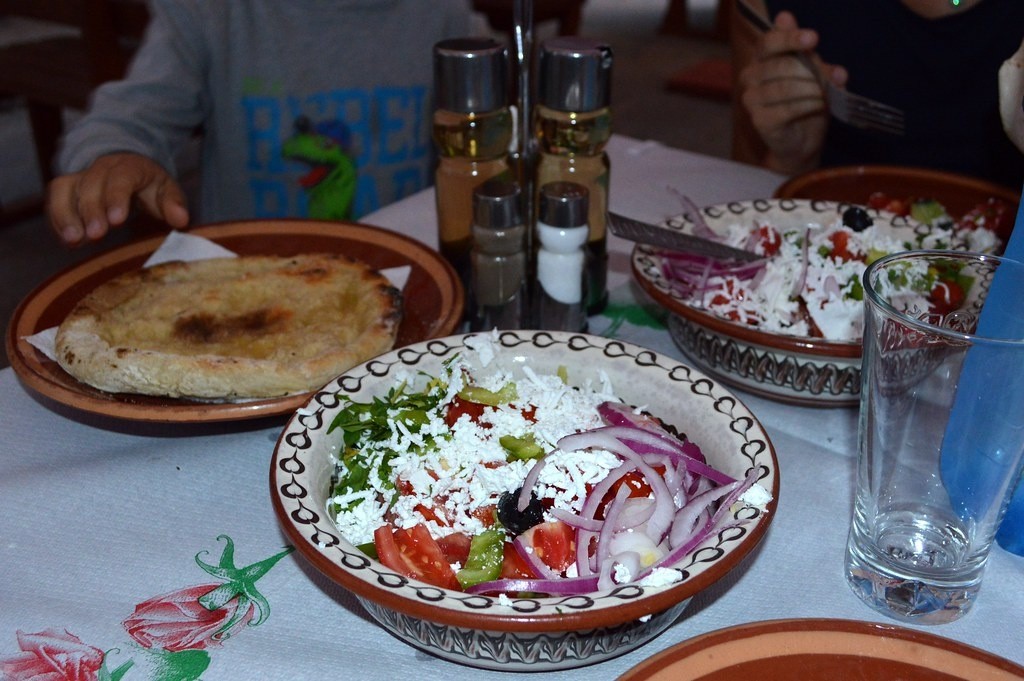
xmin=732 ymin=0 xmax=905 ymax=137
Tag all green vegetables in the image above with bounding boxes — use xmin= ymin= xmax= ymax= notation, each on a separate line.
xmin=322 ymin=352 xmax=458 ymax=514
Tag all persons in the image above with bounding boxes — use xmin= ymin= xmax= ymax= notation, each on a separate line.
xmin=49 ymin=1 xmax=510 ymax=245
xmin=728 ymin=0 xmax=1024 ymax=193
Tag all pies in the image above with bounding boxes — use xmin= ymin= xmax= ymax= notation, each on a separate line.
xmin=59 ymin=251 xmax=402 ymax=400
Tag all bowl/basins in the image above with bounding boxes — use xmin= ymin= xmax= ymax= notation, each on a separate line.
xmin=629 ymin=195 xmax=996 ymax=412
xmin=271 ymin=327 xmax=782 ymax=674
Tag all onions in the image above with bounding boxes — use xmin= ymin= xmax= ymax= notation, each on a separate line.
xmin=464 ymin=405 xmax=759 ymax=596
xmin=663 ymin=189 xmax=769 ymax=305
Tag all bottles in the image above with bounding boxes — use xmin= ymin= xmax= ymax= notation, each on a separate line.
xmin=429 ymin=34 xmax=523 ymax=320
xmin=529 ymin=35 xmax=616 ymax=316
xmin=467 ymin=180 xmax=527 ymax=341
xmin=533 ymin=177 xmax=593 ymax=335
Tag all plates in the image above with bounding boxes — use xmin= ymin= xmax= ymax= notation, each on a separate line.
xmin=774 ymin=163 xmax=1020 ymax=271
xmin=613 ymin=614 xmax=1024 ymax=681
xmin=4 ymin=216 xmax=464 ymax=427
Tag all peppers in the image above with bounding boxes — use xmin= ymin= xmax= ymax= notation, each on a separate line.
xmin=454 ymin=509 xmax=504 ymax=590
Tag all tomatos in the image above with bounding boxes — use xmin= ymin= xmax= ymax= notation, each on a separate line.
xmin=377 ymin=395 xmax=654 ymax=589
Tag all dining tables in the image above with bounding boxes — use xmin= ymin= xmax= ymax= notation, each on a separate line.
xmin=0 ymin=91 xmax=1024 ymax=681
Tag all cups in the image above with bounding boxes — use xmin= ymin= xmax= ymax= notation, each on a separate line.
xmin=841 ymin=244 xmax=1023 ymax=630
